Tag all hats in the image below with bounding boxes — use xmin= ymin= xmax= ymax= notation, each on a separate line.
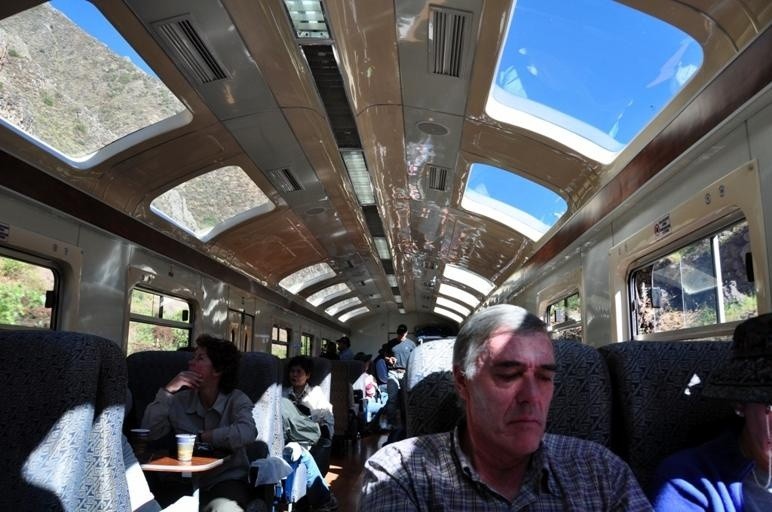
xmin=702 ymin=313 xmax=771 ymax=405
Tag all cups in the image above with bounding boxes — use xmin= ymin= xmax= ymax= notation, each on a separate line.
xmin=175 ymin=434 xmax=197 ymax=462
xmin=130 ymin=429 xmax=151 ymax=463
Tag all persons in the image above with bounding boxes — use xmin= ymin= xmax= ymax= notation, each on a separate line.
xmin=326 ymin=324 xmax=417 ymax=443
xmin=658 ymin=313 xmax=772 ymax=512
xmin=142 ymin=335 xmax=258 ymax=511
xmin=355 ymin=302 xmax=655 ymax=512
xmin=280 ymin=359 xmax=341 ymax=512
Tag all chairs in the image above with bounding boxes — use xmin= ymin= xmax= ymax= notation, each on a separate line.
xmin=410 ymin=328 xmax=747 ymax=486
xmin=124 ymin=343 xmax=370 ymax=471
xmin=0 ymin=326 xmax=155 ymax=512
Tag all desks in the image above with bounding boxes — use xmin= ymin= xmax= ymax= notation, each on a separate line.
xmin=133 ymin=450 xmax=231 ymax=499
